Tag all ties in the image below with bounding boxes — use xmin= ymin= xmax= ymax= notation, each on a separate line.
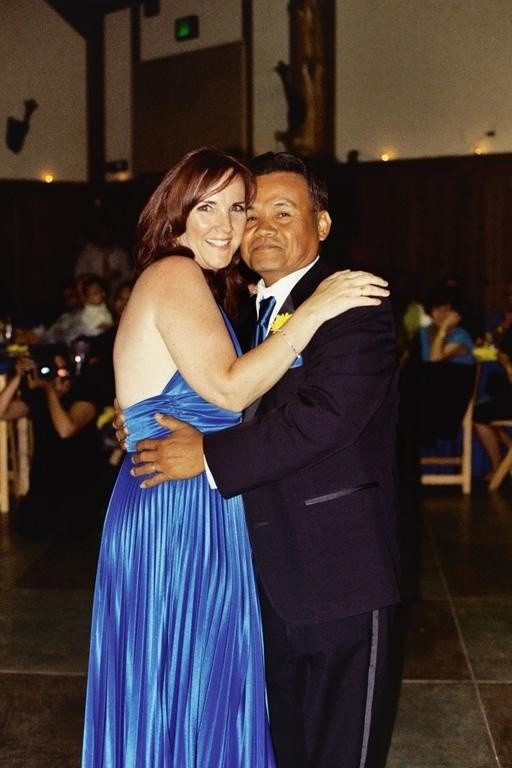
xmin=249 ymin=295 xmax=277 ymax=349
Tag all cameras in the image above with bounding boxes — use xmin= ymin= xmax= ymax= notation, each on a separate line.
xmin=27 ymin=363 xmax=59 ymax=381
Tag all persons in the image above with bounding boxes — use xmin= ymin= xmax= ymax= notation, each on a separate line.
xmin=108 ymin=152 xmax=416 ymax=768
xmin=77 ymin=143 xmax=393 ymax=768
xmin=397 ymin=287 xmax=512 ymax=490
xmin=0 ymin=276 xmax=135 ymax=541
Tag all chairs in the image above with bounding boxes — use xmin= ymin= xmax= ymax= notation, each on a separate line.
xmin=418 ymin=353 xmax=512 ymax=496
